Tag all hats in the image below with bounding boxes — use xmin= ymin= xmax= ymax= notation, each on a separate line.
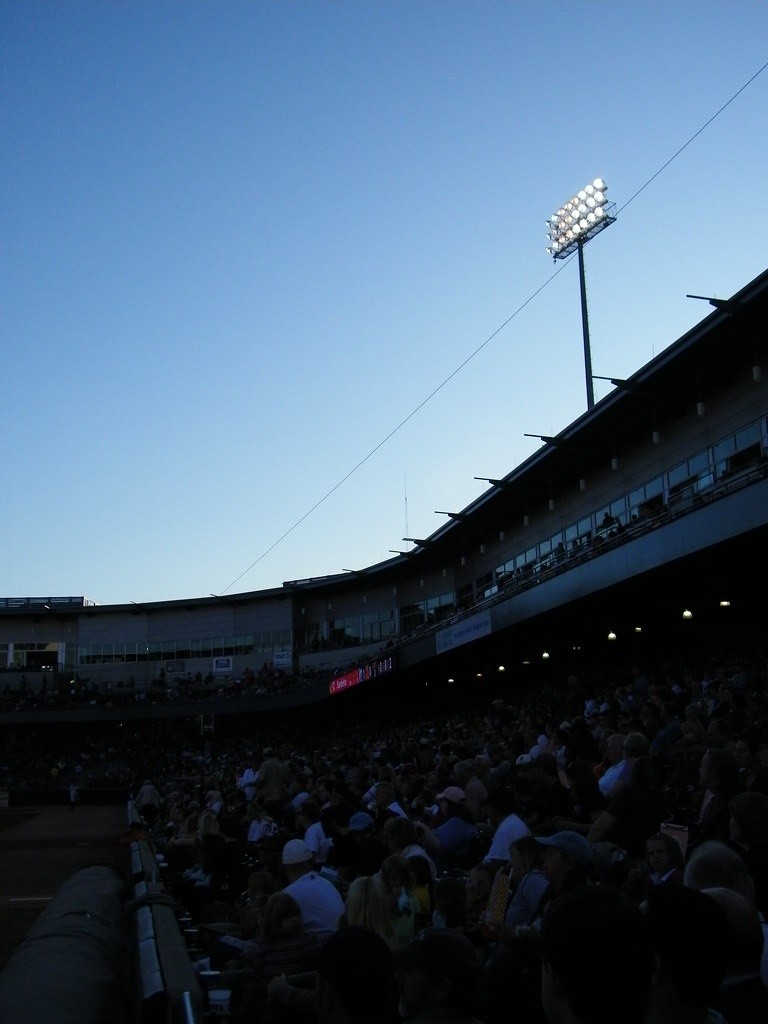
xmin=282 ymin=838 xmax=313 ymax=864
xmin=349 ymin=812 xmax=374 ymax=831
xmin=263 ymin=748 xmax=273 ymax=753
xmin=516 ymin=753 xmax=534 ymax=765
xmin=623 ymin=733 xmax=650 ymax=746
xmin=436 ymin=787 xmax=465 ymax=804
xmin=534 ymin=830 xmax=592 ymax=870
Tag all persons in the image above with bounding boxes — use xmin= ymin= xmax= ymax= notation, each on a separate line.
xmin=0 ymin=455 xmax=768 ymax=1024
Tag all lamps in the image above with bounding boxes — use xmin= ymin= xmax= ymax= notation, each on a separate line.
xmin=611 ymin=459 xmax=619 ymax=470
xmin=499 ymin=532 xmax=504 ymax=542
xmin=653 ymin=432 xmax=659 ymax=444
xmin=753 ymin=366 xmax=762 ymax=384
xmin=580 ymin=480 xmax=587 ymax=491
xmin=523 ymin=517 xmax=529 ymax=527
xmin=460 ymin=557 xmax=465 ymax=566
xmin=480 ymin=545 xmax=486 ymax=553
xmin=443 ymin=570 xmax=447 ymax=576
xmin=697 ymin=403 xmax=705 ymax=416
xmin=548 ymin=499 xmax=555 ymax=511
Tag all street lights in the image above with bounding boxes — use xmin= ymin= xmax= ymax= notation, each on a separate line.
xmin=545 ymin=178 xmax=617 ymax=411
